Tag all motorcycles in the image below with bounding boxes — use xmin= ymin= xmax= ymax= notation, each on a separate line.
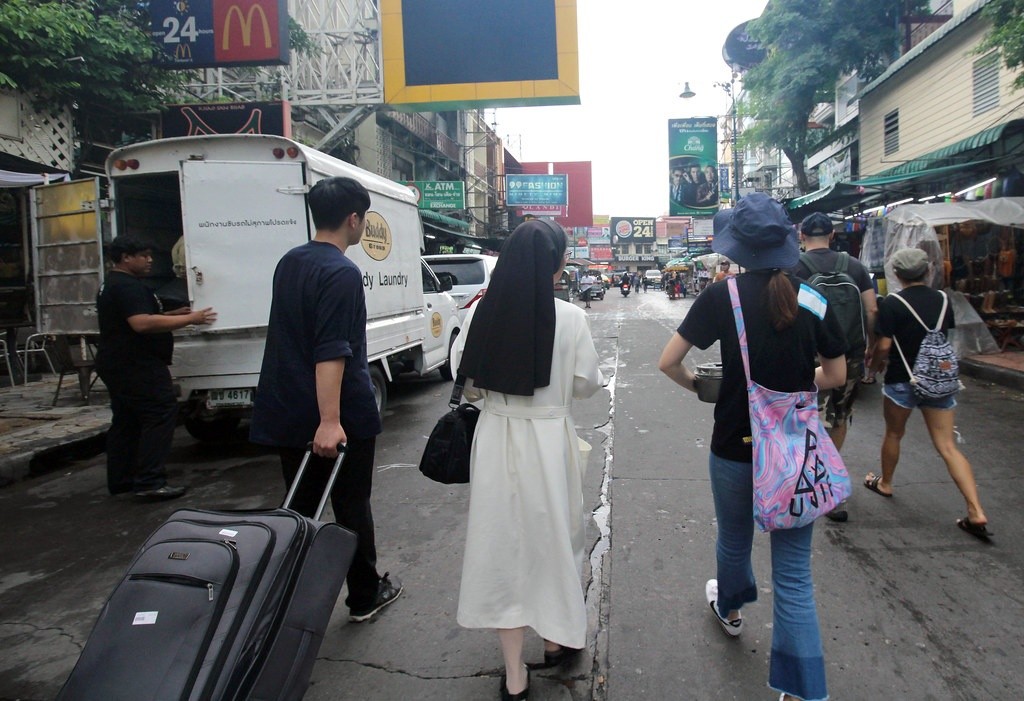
xmin=619 ymin=280 xmax=631 ymax=297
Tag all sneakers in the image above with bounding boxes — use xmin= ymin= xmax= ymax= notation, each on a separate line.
xmin=778 ymin=693 xmax=785 ymax=701
xmin=348 ymin=572 xmax=403 ymax=623
xmin=706 ymin=579 xmax=743 ymax=638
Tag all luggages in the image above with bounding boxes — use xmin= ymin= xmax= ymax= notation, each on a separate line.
xmin=51 ymin=437 xmax=358 ymax=701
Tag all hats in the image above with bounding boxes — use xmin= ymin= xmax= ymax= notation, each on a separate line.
xmin=710 ymin=190 xmax=799 ymax=272
xmin=892 ymin=248 xmax=929 ymax=280
xmin=799 ymin=212 xmax=832 ymax=237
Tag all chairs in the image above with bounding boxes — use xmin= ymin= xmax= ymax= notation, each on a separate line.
xmin=15 ymin=333 xmax=57 ymax=388
xmin=0 ymin=331 xmax=16 ymax=388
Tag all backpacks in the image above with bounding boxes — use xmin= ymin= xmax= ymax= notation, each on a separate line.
xmin=886 ymin=287 xmax=966 ymax=398
xmin=794 ymin=249 xmax=867 ymax=356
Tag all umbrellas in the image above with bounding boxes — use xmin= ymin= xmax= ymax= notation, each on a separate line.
xmin=661 ymin=264 xmax=690 ymax=271
xmin=567 ymin=258 xmax=596 ymax=265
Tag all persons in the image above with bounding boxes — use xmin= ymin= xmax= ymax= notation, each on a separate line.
xmin=451 ymin=220 xmax=606 ymax=701
xmin=657 ymin=191 xmax=846 ymax=701
xmin=93 ymin=232 xmax=217 ymax=501
xmin=667 ymin=272 xmax=686 ymax=300
xmin=251 ymin=176 xmax=404 ymax=622
xmin=863 ymin=245 xmax=996 ymax=539
xmin=791 ymin=210 xmax=877 ymax=522
xmin=714 ymin=260 xmax=736 ymax=282
xmin=670 ymin=163 xmax=717 ymax=206
xmin=610 ymin=271 xmax=649 ymax=294
xmin=579 ymin=270 xmax=594 ymax=308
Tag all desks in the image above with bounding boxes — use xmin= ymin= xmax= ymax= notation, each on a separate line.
xmin=989 ymin=320 xmax=1024 ymax=352
xmin=0 ymin=322 xmax=41 ymax=385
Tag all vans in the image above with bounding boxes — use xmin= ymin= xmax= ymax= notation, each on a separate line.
xmin=423 ymin=255 xmax=498 ymax=326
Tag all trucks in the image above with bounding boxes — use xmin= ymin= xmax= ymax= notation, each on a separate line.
xmin=29 ymin=135 xmax=465 ymax=446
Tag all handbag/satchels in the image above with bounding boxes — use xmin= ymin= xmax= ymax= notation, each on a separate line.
xmin=418 ymin=402 xmax=480 ymax=486
xmin=742 ymin=380 xmax=854 ymax=533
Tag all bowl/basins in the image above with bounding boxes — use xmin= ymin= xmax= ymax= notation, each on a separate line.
xmin=694 ymin=361 xmax=722 ymax=403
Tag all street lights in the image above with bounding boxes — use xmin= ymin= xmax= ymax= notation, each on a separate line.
xmin=681 ymin=81 xmax=741 ymax=205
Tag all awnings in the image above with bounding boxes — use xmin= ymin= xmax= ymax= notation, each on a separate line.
xmin=419 ymin=209 xmax=472 ymax=229
xmin=786 ymin=181 xmax=855 ymax=210
xmin=849 ymin=117 xmax=1024 ymax=186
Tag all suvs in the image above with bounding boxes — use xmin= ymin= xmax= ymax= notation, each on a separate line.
xmin=644 ymin=269 xmax=663 ymax=283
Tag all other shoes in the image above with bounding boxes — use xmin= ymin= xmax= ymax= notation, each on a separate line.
xmin=543 ymin=642 xmax=568 ymax=665
xmin=131 ymin=484 xmax=187 ymax=503
xmin=500 ymin=666 xmax=530 ymax=701
xmin=826 ymin=509 xmax=848 ymax=522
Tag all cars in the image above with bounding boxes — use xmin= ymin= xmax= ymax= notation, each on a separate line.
xmin=579 ymin=281 xmax=605 ymax=301
xmin=605 ymin=272 xmax=637 ymax=286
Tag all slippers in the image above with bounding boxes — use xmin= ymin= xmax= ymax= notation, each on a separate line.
xmin=957 ymin=517 xmax=995 ymax=536
xmin=863 ymin=474 xmax=892 ymax=498
